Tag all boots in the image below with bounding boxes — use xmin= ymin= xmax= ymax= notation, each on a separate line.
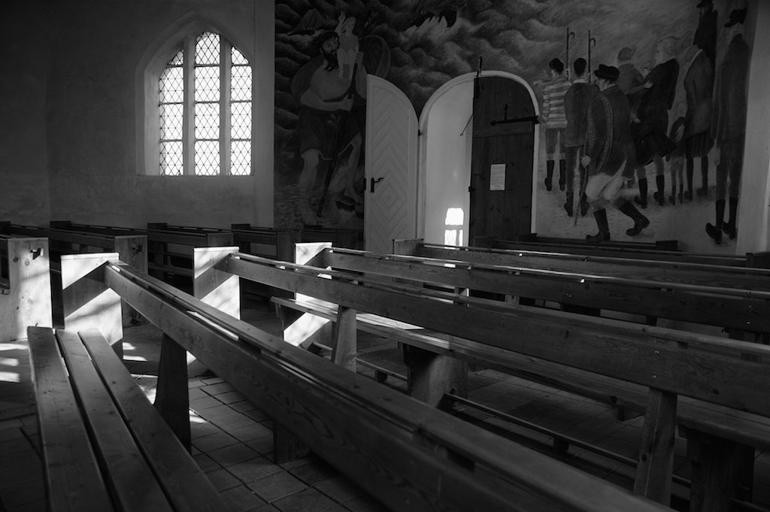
xmin=706 ymin=199 xmax=725 ymax=244
xmin=619 ymin=201 xmax=649 ymax=236
xmin=559 ymin=159 xmax=566 ymax=190
xmin=670 ymin=180 xmax=692 ymax=204
xmin=579 ymin=191 xmax=588 ymax=216
xmin=634 ymin=178 xmax=647 ymax=208
xmin=545 ymin=160 xmax=554 ymax=190
xmin=723 ymin=196 xmax=737 ymax=239
xmin=564 ymin=192 xmax=573 ymax=216
xmin=654 ymin=175 xmax=664 ymax=205
xmin=586 ymin=208 xmax=610 ymax=242
xmin=697 ymin=177 xmax=707 ymax=195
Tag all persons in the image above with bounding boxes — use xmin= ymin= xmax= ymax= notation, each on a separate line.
xmin=283 ymin=30 xmax=368 ymax=228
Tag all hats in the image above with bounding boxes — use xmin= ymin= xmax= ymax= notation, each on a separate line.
xmin=723 ymin=9 xmax=747 ymax=28
xmin=695 ymin=9 xmax=713 ymax=46
xmin=593 ymin=64 xmax=619 ymax=80
